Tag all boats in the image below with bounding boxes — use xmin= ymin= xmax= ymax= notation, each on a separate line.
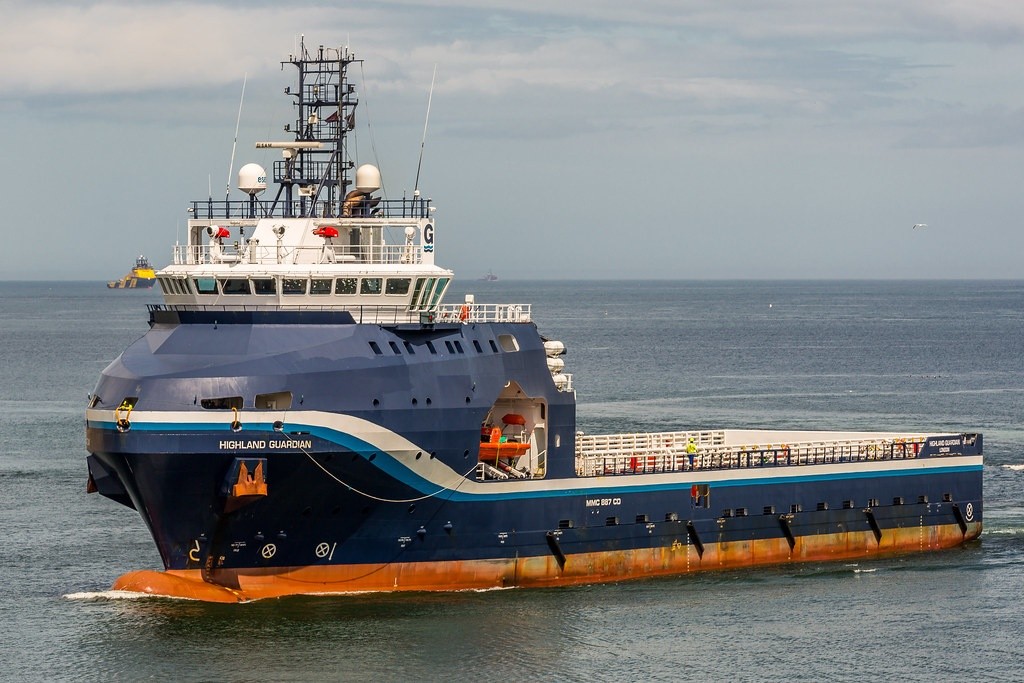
xmin=105 ymin=255 xmax=156 ymax=289
xmin=478 ymin=413 xmax=530 ymax=458
xmin=82 ymin=38 xmax=985 ymax=607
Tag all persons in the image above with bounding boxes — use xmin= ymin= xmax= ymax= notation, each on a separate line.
xmin=686 ymin=437 xmax=698 ymax=470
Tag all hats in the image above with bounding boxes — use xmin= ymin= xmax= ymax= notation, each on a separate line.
xmin=690 ymin=437 xmax=694 ymax=441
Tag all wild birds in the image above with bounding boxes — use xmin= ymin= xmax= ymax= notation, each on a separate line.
xmin=605 ymin=309 xmax=609 ymax=316
xmin=769 ymin=303 xmax=773 ymax=309
xmin=912 ymin=223 xmax=931 ymax=233
xmin=845 ymin=373 xmax=950 ymax=398
